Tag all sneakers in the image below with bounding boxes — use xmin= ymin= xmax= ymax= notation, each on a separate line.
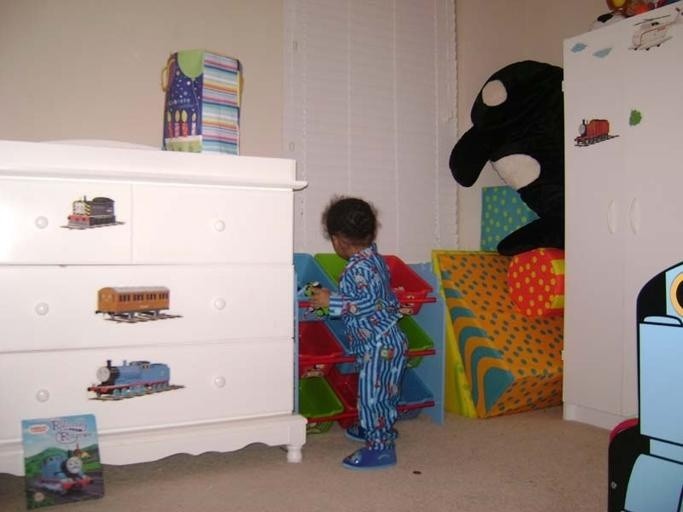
xmin=342 ymin=447 xmax=397 ymax=471
xmin=346 ymin=424 xmax=398 ymax=441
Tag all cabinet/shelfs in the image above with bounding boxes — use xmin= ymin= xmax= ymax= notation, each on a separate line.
xmin=0 ymin=138 xmax=308 ymax=476
xmin=293 ymin=253 xmax=445 ymax=434
xmin=561 ymin=0 xmax=683 ymax=430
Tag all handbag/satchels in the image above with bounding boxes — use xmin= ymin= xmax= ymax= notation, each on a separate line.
xmin=160 ymin=49 xmax=243 ymax=153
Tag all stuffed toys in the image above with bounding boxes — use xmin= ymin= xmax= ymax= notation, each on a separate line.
xmin=449 ymin=60 xmax=566 ymax=257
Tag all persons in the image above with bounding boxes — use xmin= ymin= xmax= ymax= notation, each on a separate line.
xmin=307 ymin=197 xmax=408 ymax=469
